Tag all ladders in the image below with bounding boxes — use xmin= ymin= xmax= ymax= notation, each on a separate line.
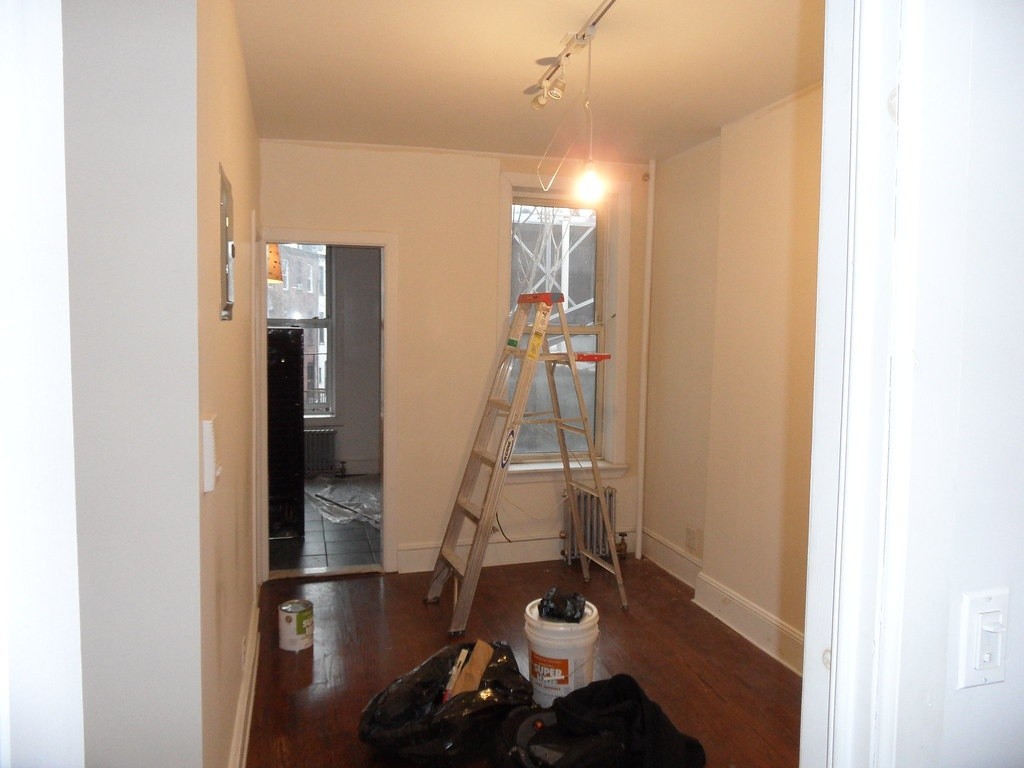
xmin=423 ymin=292 xmax=630 ymax=637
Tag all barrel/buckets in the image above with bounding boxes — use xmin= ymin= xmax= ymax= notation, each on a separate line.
xmin=524 ymin=597 xmax=600 ymax=708
xmin=278 ymin=599 xmax=314 ymax=651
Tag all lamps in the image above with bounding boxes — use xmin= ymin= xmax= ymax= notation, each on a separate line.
xmin=266 ymin=243 xmax=282 ymax=284
xmin=529 ymin=63 xmax=567 ymax=109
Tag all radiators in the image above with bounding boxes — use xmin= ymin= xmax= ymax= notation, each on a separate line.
xmin=306 ymin=429 xmax=338 ymax=474
xmin=563 ymin=485 xmax=616 ymax=567
